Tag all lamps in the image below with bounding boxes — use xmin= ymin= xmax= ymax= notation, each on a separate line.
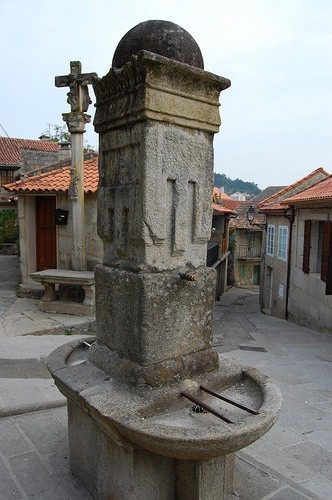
xmin=247 ymin=206 xmax=255 ymax=221
